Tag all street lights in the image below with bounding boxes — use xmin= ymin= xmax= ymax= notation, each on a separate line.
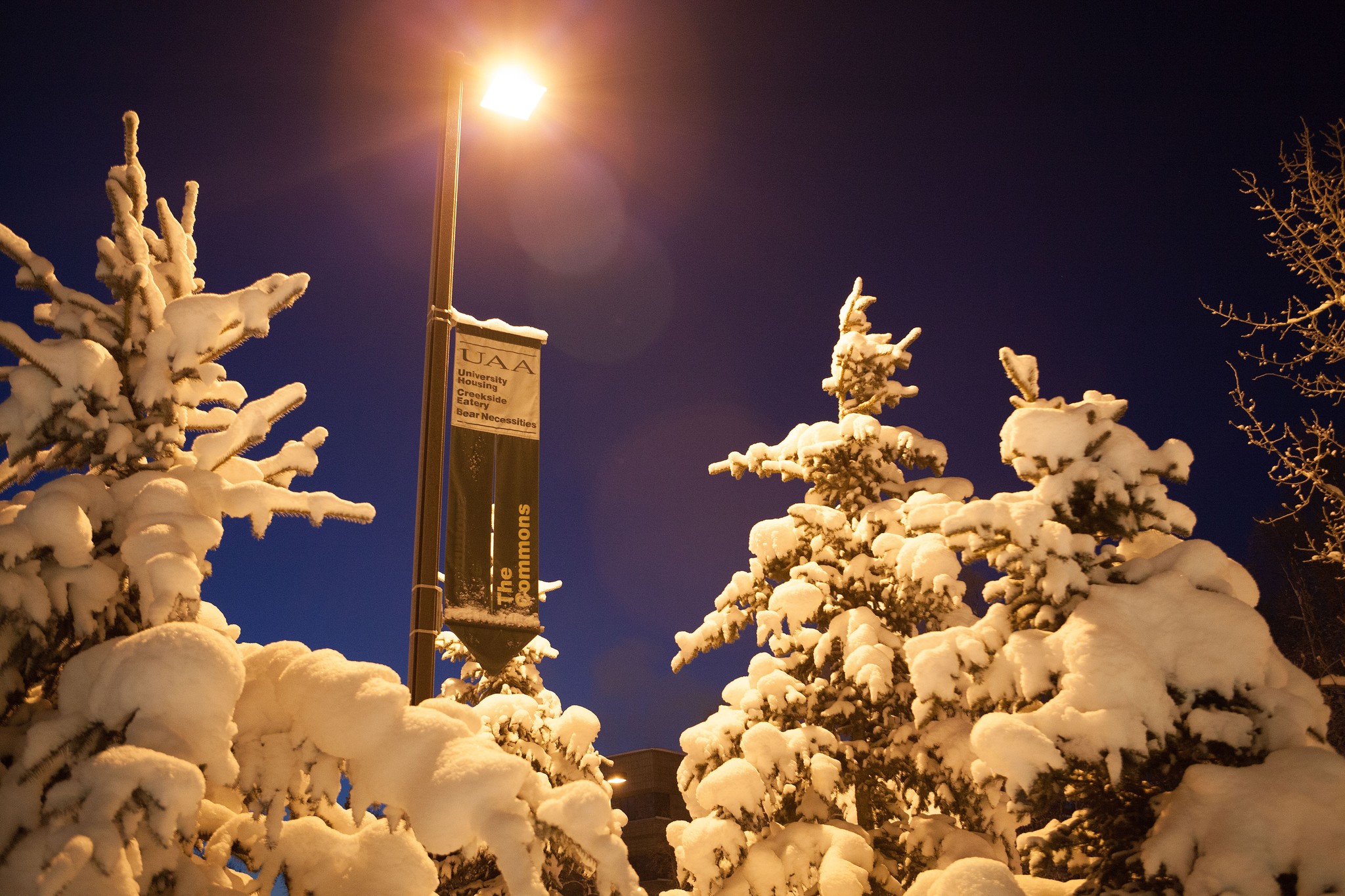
xmin=408 ymin=43 xmax=546 ymax=704
